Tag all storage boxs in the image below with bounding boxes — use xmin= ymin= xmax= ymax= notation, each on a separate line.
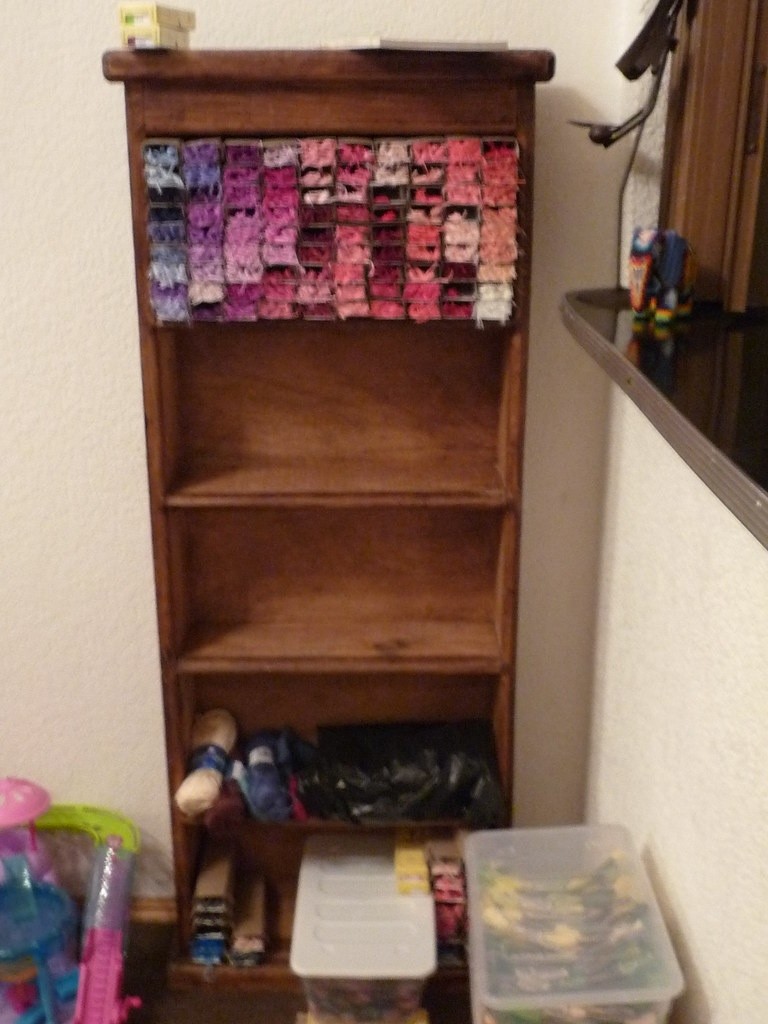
xmin=288 ymin=831 xmax=441 ymax=1024
xmin=462 ymin=823 xmax=688 ymax=1024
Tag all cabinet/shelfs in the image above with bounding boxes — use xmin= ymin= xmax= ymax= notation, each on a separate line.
xmin=100 ymin=44 xmax=560 ymax=1024
